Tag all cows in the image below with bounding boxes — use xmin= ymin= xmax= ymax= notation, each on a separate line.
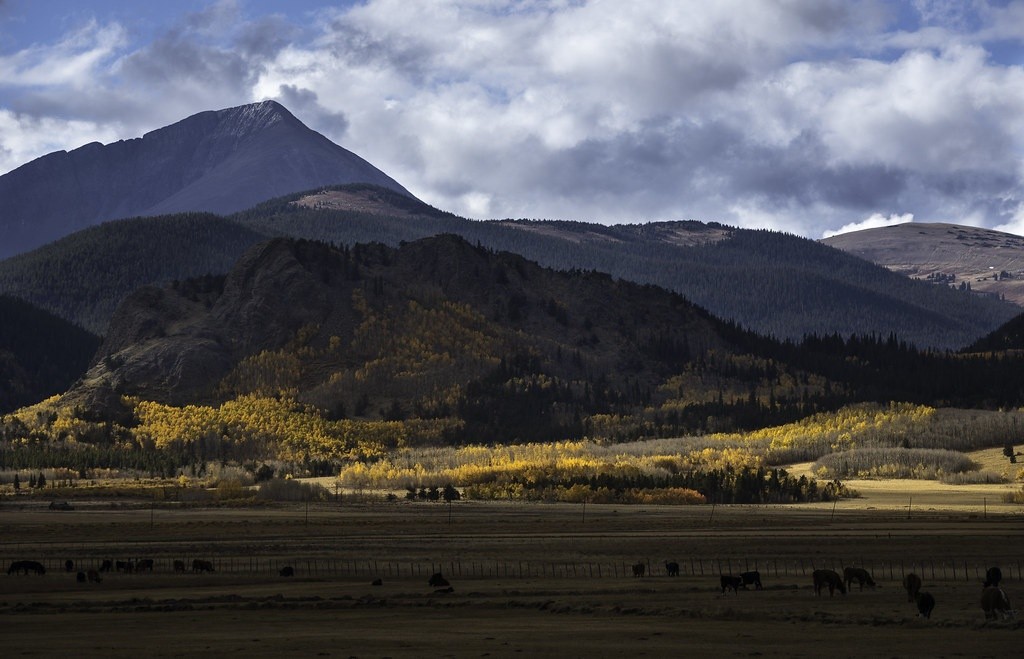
xmin=632 ymin=563 xmax=645 ymax=578
xmin=429 ymin=572 xmax=454 ymax=593
xmin=135 ymin=559 xmax=154 ymax=575
xmin=813 ymin=569 xmax=845 ymax=599
xmin=77 ymin=572 xmax=86 ymax=583
xmin=6 ymin=561 xmax=46 ymax=577
xmin=981 ymin=587 xmax=1015 ymax=622
xmin=843 ymin=567 xmax=876 ymax=592
xmin=739 ymin=571 xmax=762 ymax=591
xmin=65 ymin=560 xmax=74 ymax=572
xmin=192 ymin=559 xmax=214 ymax=574
xmin=720 ymin=576 xmax=742 ymax=596
xmin=279 ymin=567 xmax=295 ymax=577
xmin=666 ymin=560 xmax=679 ymax=577
xmin=984 ymin=567 xmax=1003 ymax=586
xmin=915 ymin=591 xmax=935 ymax=620
xmin=87 ymin=569 xmax=103 ymax=583
xmin=115 ymin=560 xmax=134 ymax=575
xmin=903 ymin=574 xmax=921 ymax=603
xmin=173 ymin=560 xmax=185 ymax=572
xmin=99 ymin=560 xmax=114 ymax=574
